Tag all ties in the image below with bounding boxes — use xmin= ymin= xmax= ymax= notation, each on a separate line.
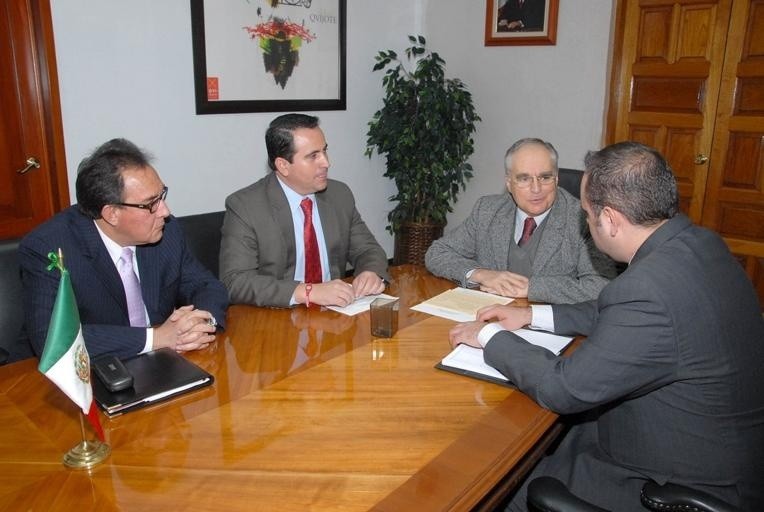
xmin=120 ymin=248 xmax=146 ymax=327
xmin=517 ymin=217 xmax=537 ymax=247
xmin=300 ymin=198 xmax=323 ymax=284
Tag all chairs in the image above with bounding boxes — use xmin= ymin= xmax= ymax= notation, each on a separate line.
xmin=175 ymin=212 xmax=224 ymax=279
xmin=555 ymin=168 xmax=585 ymax=200
xmin=0 ymin=236 xmax=27 ymax=366
xmin=519 ymin=470 xmax=760 ymax=510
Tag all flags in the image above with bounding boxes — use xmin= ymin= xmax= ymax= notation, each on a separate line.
xmin=42 ymin=253 xmax=105 ymax=444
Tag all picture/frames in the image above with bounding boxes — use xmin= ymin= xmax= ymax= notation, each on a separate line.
xmin=481 ymin=0 xmax=560 ymax=49
xmin=190 ymin=0 xmax=351 ymax=118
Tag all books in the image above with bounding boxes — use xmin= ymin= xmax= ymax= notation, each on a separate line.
xmin=437 ymin=314 xmax=576 ymax=385
xmin=84 ymin=348 xmax=214 ymax=416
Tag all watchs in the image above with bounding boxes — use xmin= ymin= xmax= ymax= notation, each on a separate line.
xmin=304 ymin=283 xmax=312 ymax=308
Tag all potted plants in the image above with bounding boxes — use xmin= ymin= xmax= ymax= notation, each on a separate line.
xmin=361 ymin=29 xmax=483 ymax=267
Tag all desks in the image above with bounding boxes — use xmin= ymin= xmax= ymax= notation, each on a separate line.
xmin=0 ymin=258 xmax=619 ymax=510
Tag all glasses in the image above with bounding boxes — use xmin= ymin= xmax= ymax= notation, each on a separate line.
xmin=511 ymin=175 xmax=556 ymax=188
xmin=113 ymin=186 xmax=168 ymax=214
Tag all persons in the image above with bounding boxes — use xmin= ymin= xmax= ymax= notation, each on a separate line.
xmin=224 ymin=306 xmax=360 ymax=415
xmin=217 ymin=112 xmax=395 ymax=308
xmin=442 ymin=145 xmax=763 ymax=511
xmin=13 ymin=138 xmax=234 ymax=362
xmin=452 ymin=374 xmax=541 ymax=429
xmin=103 ymin=405 xmax=192 ymax=495
xmin=421 ymin=136 xmax=616 ymax=306
xmin=493 ymin=0 xmax=545 ymax=31
